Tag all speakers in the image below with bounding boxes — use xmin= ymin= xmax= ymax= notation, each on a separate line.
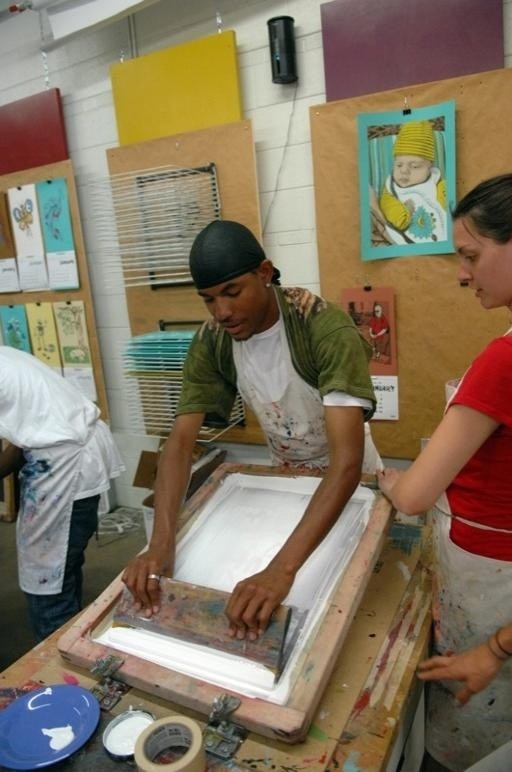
xmin=267 ymin=15 xmax=298 ymax=83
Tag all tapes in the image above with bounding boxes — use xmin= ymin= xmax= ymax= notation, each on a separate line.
xmin=134 ymin=715 xmax=203 ymax=772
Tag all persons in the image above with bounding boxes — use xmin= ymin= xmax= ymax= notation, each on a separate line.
xmin=120 ymin=220 xmax=388 ymax=645
xmin=376 ymin=174 xmax=512 ymax=772
xmin=0 ymin=345 xmax=126 ymax=644
xmin=379 ymin=120 xmax=448 ymax=243
xmin=367 ymin=305 xmax=388 ymax=357
xmin=416 ymin=624 xmax=512 ymax=707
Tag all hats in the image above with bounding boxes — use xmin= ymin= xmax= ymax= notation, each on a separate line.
xmin=188 ymin=216 xmax=266 ymax=290
xmin=391 ymin=120 xmax=437 ymax=161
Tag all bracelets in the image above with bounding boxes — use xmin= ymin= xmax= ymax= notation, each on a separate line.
xmin=487 ymin=637 xmax=508 ymax=663
xmin=496 ymin=627 xmax=512 ymax=657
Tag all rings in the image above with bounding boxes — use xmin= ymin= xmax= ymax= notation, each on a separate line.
xmin=148 ymin=572 xmax=161 ymax=584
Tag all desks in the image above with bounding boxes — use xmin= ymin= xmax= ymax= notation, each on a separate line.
xmin=0 ymin=520 xmax=435 ymax=772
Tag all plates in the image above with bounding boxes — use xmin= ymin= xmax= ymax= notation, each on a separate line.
xmin=0 ymin=685 xmax=101 ymax=769
